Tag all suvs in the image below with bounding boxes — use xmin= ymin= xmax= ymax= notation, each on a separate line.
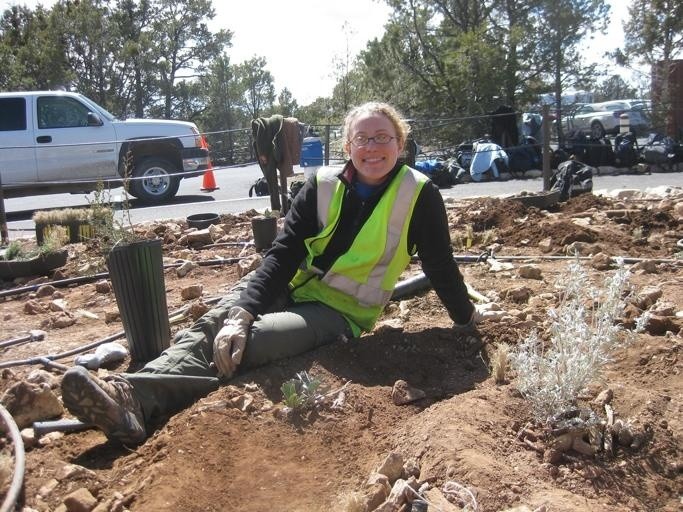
xmin=0 ymin=89 xmax=209 ymax=205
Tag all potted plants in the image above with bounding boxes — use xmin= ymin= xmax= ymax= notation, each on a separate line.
xmin=0 ymin=241 xmax=68 ymax=277
xmin=85 ymin=144 xmax=171 ymax=361
xmin=33 ymin=208 xmax=113 ymax=246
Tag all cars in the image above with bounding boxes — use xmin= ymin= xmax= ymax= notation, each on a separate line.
xmin=551 ymin=98 xmax=663 ymax=141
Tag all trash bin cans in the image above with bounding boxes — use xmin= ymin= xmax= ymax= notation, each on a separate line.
xmin=299 ymin=137 xmax=323 ymax=166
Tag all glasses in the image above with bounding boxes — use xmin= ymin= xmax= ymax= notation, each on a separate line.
xmin=347 ymin=133 xmax=399 ymax=147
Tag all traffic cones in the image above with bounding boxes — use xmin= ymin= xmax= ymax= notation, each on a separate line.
xmin=200 ymin=160 xmax=219 ymax=191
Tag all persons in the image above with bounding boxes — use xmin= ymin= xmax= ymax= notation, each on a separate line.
xmin=56 ymin=99 xmax=511 ymax=448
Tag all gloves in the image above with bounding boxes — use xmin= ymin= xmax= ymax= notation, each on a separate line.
xmin=454 ymin=302 xmax=508 ymax=329
xmin=212 ymin=307 xmax=254 ymax=380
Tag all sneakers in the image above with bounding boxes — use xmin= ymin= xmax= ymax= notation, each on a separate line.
xmin=60 ymin=365 xmax=147 ymax=445
